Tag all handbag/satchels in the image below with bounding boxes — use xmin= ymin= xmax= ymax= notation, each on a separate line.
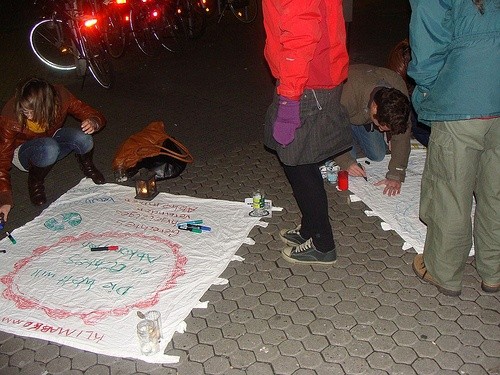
xmin=112 ymin=121 xmax=194 ymax=174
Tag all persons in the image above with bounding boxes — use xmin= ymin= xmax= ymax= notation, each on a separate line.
xmin=329 ymin=63 xmax=413 ymax=196
xmin=261 ymin=0 xmax=354 ymax=266
xmin=0 ymin=76 xmax=107 ymax=222
xmin=407 ymin=0 xmax=500 ymax=297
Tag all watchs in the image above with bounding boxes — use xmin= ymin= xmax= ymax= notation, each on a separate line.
xmin=89 ymin=119 xmax=100 ymax=130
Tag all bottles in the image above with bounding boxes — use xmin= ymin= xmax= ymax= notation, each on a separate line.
xmin=136 ymin=319 xmax=161 ymax=357
xmin=252 ymin=189 xmax=265 ymax=217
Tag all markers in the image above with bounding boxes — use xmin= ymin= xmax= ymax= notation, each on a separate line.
xmin=91 ymin=246 xmax=118 ymax=251
xmin=358 ymin=163 xmax=367 ymax=181
xmin=186 ymin=224 xmax=211 ymax=231
xmin=177 ymin=220 xmax=203 ymax=226
xmin=6 ymin=231 xmax=16 ymax=244
xmin=178 ymin=226 xmax=202 ymax=233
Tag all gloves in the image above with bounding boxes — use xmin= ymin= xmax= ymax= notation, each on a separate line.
xmin=273 ymin=95 xmax=303 ymax=146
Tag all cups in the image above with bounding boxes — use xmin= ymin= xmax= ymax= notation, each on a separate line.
xmin=144 ymin=310 xmax=163 ymax=340
xmin=113 ymin=164 xmax=127 ymax=184
xmin=326 ymin=166 xmax=340 ymax=184
xmin=337 ymin=170 xmax=349 ymax=190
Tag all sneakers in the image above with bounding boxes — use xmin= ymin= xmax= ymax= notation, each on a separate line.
xmin=412 ymin=254 xmax=461 ymax=297
xmin=481 ymin=281 xmax=500 ymax=293
xmin=279 ymin=224 xmax=307 ymax=247
xmin=280 ymin=236 xmax=337 ymax=265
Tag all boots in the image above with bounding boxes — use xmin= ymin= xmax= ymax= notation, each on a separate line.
xmin=27 ymin=163 xmax=56 ymax=206
xmin=74 ymin=143 xmax=106 ymax=185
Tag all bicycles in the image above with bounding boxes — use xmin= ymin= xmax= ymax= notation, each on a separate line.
xmin=27 ymin=0 xmax=259 ymax=91
